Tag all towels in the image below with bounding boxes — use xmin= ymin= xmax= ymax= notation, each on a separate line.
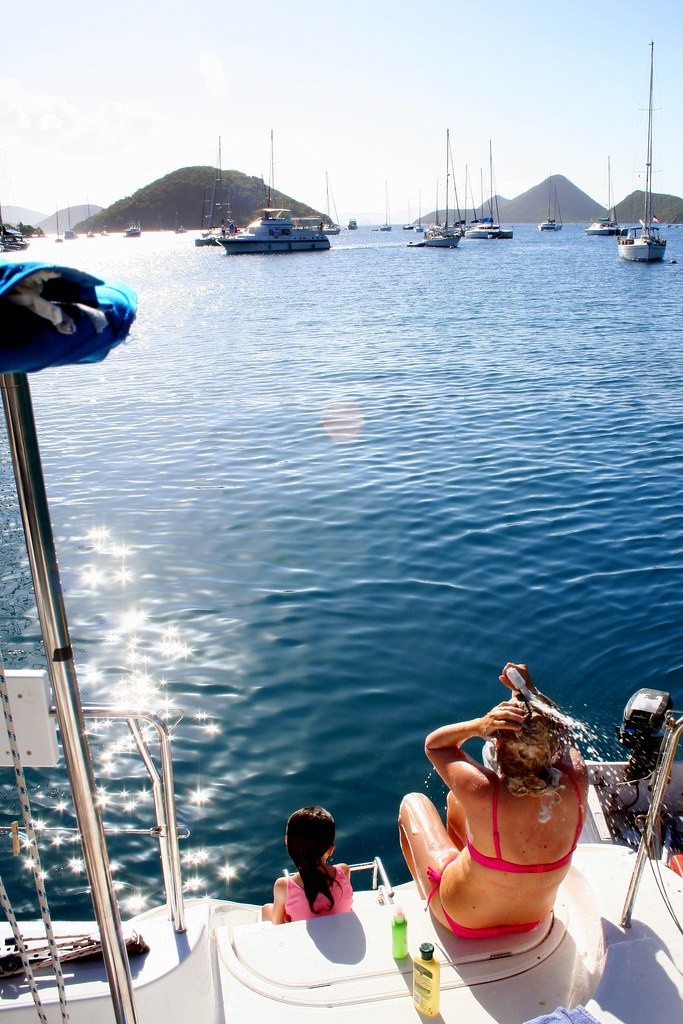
xmin=521 ymin=1003 xmax=603 ymax=1024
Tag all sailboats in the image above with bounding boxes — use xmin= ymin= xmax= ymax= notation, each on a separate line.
xmin=617 ymin=38 xmax=667 ymax=262
xmin=421 ymin=128 xmax=513 ymax=247
xmin=538 ymin=171 xmax=563 ymax=231
xmin=380 ymin=181 xmax=392 ymax=231
xmin=416 ymin=196 xmax=424 ymax=232
xmin=194 ymin=135 xmax=238 ymax=245
xmin=239 ymin=129 xmax=342 ymax=236
xmin=402 ymin=198 xmax=414 ymax=230
xmin=584 ymin=155 xmax=626 ymax=235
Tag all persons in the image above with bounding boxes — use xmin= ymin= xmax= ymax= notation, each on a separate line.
xmin=320 ymin=222 xmax=324 ymax=231
xmin=230 ymin=223 xmax=237 ymax=235
xmin=398 ymin=662 xmax=589 ymax=939
xmin=221 ymin=224 xmax=226 ymax=236
xmin=262 ymin=805 xmax=353 ymax=925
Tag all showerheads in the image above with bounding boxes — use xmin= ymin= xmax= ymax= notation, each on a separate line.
xmin=506 ymin=666 xmax=527 ymax=691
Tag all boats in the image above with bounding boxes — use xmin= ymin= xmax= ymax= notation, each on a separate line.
xmin=175 ymin=209 xmax=188 ymax=234
xmin=348 ymin=218 xmax=358 ymax=230
xmin=54 ymin=199 xmax=109 ymax=243
xmin=216 ymin=208 xmax=330 ymax=255
xmin=125 ymin=217 xmax=141 ymax=237
xmin=372 ymin=228 xmax=379 ymax=231
xmin=407 ymin=241 xmax=426 ymax=248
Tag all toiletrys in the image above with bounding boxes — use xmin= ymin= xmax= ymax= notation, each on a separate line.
xmin=412 ymin=942 xmax=441 ymax=1018
xmin=390 ymin=904 xmax=409 ymax=959
